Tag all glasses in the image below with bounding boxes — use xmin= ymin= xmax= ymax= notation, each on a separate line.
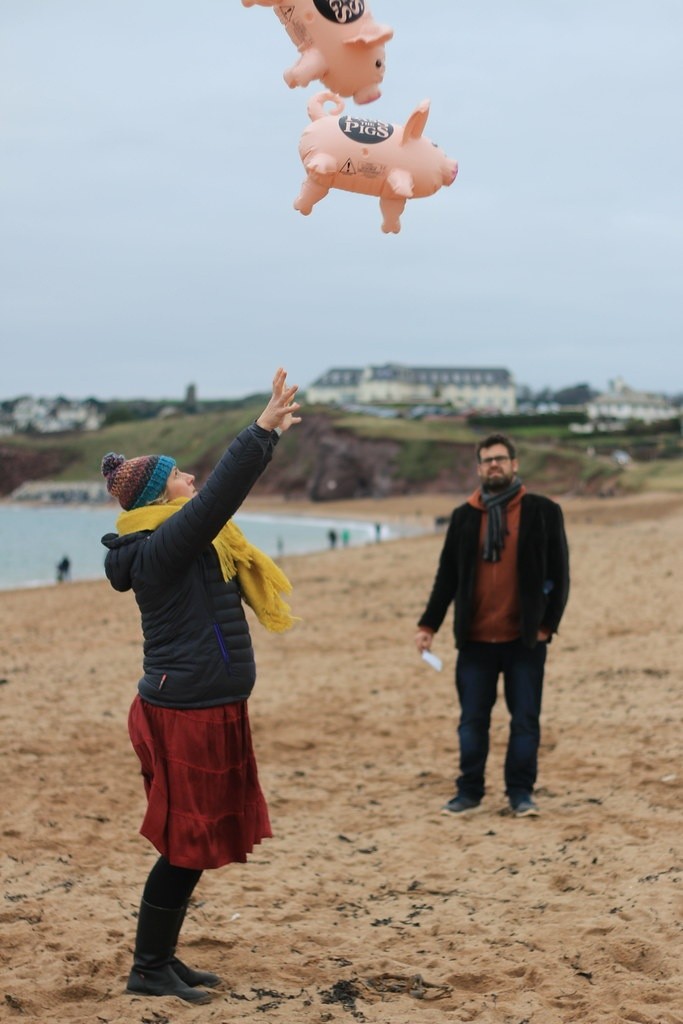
xmin=479 ymin=455 xmax=510 ymax=465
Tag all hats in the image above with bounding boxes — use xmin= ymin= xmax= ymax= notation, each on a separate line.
xmin=101 ymin=452 xmax=176 ymax=512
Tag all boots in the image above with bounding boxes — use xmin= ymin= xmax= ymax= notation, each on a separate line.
xmin=126 ymin=897 xmax=221 ymax=1005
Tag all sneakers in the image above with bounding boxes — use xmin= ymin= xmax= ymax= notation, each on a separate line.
xmin=508 ymin=795 xmax=540 ymax=818
xmin=439 ymin=796 xmax=481 ymax=816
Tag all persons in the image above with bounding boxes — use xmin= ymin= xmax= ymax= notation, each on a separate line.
xmin=101 ymin=365 xmax=305 ymax=1007
xmin=414 ymin=431 xmax=571 ymax=813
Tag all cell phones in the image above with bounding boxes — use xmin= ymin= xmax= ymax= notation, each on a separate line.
xmin=422 ymin=649 xmax=441 ymax=671
